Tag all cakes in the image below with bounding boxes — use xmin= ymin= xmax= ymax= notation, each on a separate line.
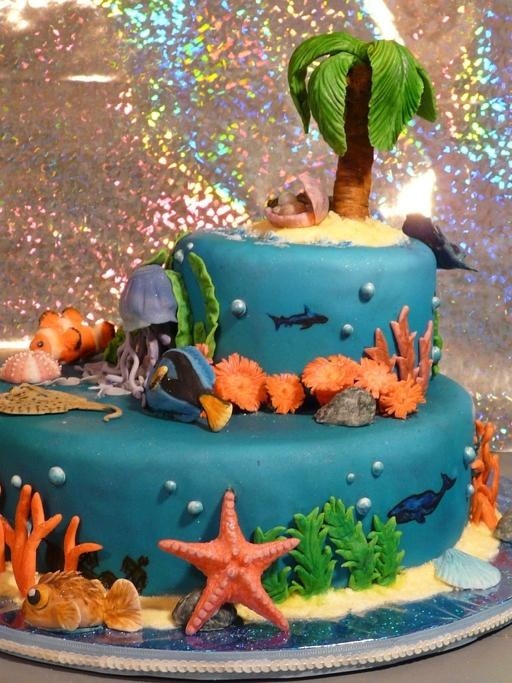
xmin=0 ymin=33 xmax=500 ymax=636
